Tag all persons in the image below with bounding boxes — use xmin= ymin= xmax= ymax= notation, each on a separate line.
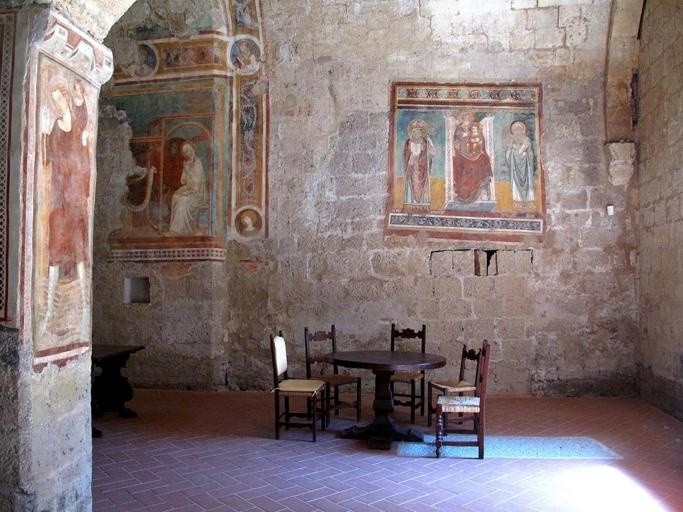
xmin=71 ymin=78 xmax=89 ymax=133
xmin=448 ymin=110 xmax=495 ymax=202
xmin=41 ymin=82 xmax=92 ymax=340
xmin=169 ymin=141 xmax=207 ymax=237
xmin=466 ymin=122 xmax=484 ymax=156
xmin=503 ymin=120 xmax=538 ymax=218
xmin=400 ymin=118 xmax=438 ymax=212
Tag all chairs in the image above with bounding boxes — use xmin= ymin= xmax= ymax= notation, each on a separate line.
xmin=270 ymin=331 xmax=325 ymax=442
xmin=428 ymin=345 xmax=481 ymax=436
xmin=436 ymin=339 xmax=491 ymax=459
xmin=388 ymin=323 xmax=426 ymax=424
xmin=304 ymin=324 xmax=361 ymax=427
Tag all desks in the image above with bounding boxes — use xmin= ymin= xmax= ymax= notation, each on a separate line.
xmin=325 ymin=351 xmax=447 ymax=450
xmin=92 ymin=344 xmax=145 ymax=438
